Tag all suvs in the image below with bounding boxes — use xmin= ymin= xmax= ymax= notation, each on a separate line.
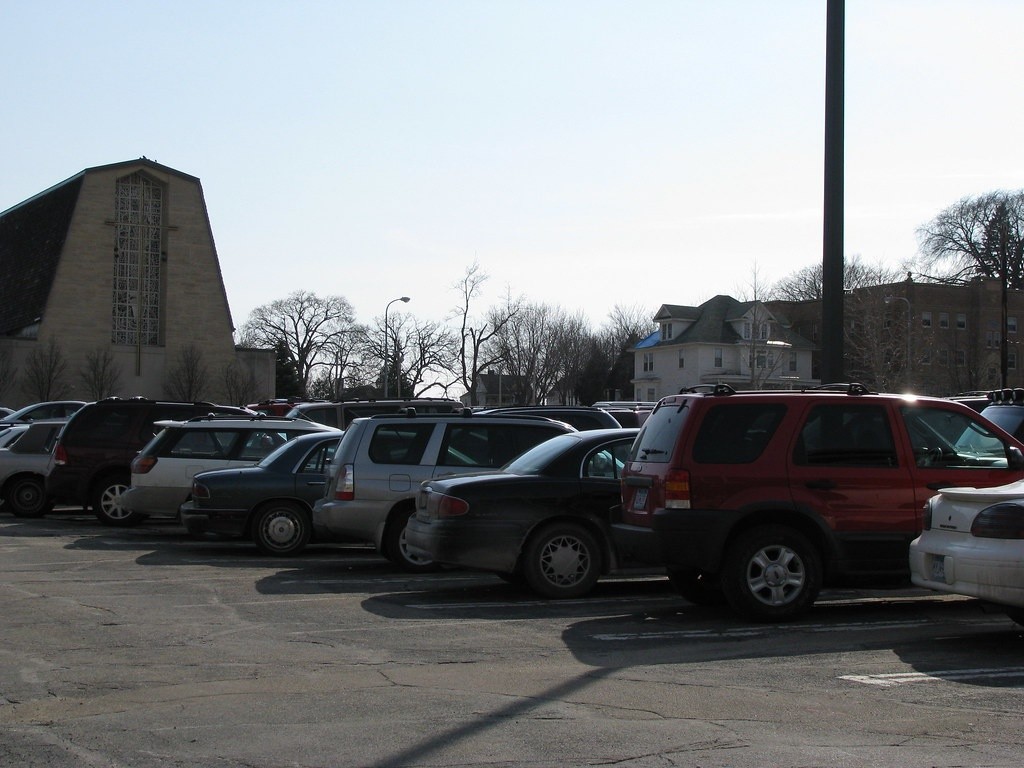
xmin=44 ymin=396 xmax=289 ymax=528
xmin=119 ymin=412 xmax=343 ymax=543
xmin=312 ymin=405 xmax=625 ymax=576
xmin=245 ymin=388 xmax=1024 ymax=467
xmin=605 ymin=383 xmax=1024 ymax=624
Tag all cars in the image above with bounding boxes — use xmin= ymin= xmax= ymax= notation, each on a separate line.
xmin=405 ymin=429 xmax=643 ymax=599
xmin=908 ymin=479 xmax=1023 ymax=630
xmin=0 ymin=401 xmax=87 ymax=518
xmin=178 ymin=429 xmax=477 ymax=558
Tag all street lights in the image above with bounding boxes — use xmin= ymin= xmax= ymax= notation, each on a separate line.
xmin=383 ymin=296 xmax=411 ymax=397
xmin=883 ymin=296 xmax=911 ymax=393
